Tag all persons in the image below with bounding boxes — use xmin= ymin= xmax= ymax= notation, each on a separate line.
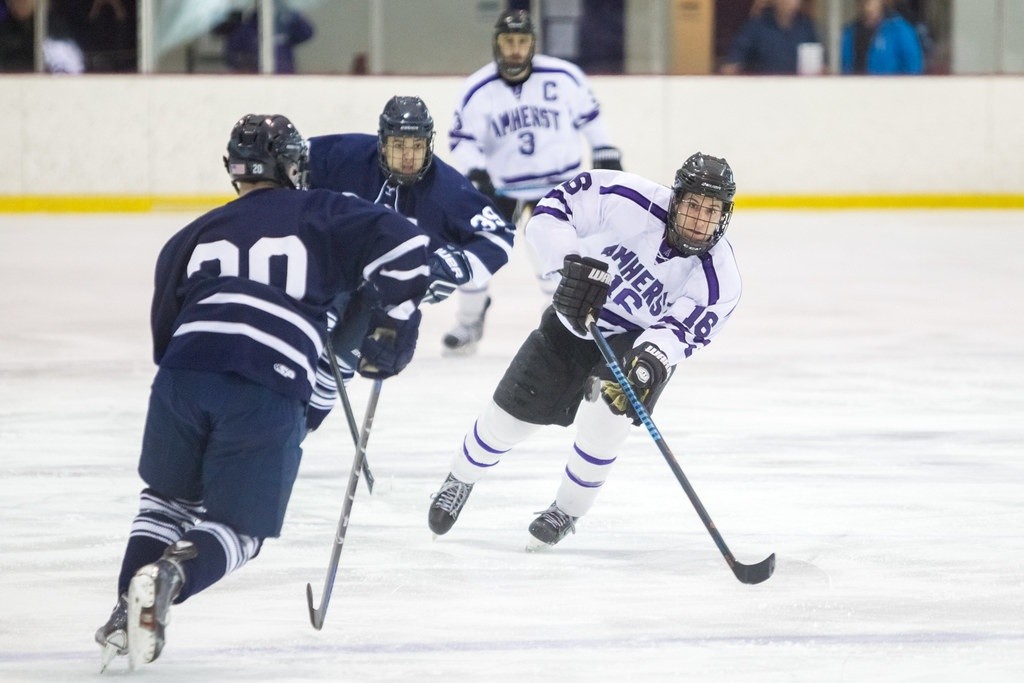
xmin=0 ymin=0 xmax=91 ymax=72
xmin=720 ymin=0 xmax=828 ymax=74
xmin=440 ymin=6 xmax=624 ymax=356
xmin=427 ymin=152 xmax=744 ymax=554
xmin=308 ymin=96 xmax=516 ymax=438
xmin=93 ymin=113 xmax=431 ymax=674
xmin=224 ymin=0 xmax=313 ymax=73
xmin=839 ymin=0 xmax=925 ymax=75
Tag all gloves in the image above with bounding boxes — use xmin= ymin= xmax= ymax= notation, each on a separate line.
xmin=603 ymin=342 xmax=669 ymax=415
xmin=418 ymin=242 xmax=472 ymax=305
xmin=357 ymin=309 xmax=422 ymax=378
xmin=552 ymin=254 xmax=612 ymax=337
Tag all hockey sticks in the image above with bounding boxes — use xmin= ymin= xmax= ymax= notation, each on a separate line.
xmin=585 ymin=312 xmax=776 ymax=585
xmin=303 ymin=330 xmax=383 ymax=628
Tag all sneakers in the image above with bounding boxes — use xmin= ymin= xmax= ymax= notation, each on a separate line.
xmin=96 ymin=593 xmax=129 ymax=674
xmin=127 ymin=542 xmax=198 ymax=663
xmin=524 ymin=502 xmax=579 ymax=551
xmin=440 ymin=297 xmax=491 ymax=360
xmin=428 ymin=473 xmax=475 ymax=542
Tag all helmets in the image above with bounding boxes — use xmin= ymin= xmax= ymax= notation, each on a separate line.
xmin=667 ymin=151 xmax=736 ymax=256
xmin=493 ymin=10 xmax=534 ymax=76
xmin=379 ymin=96 xmax=433 ymax=186
xmin=226 ymin=113 xmax=303 ymax=191
